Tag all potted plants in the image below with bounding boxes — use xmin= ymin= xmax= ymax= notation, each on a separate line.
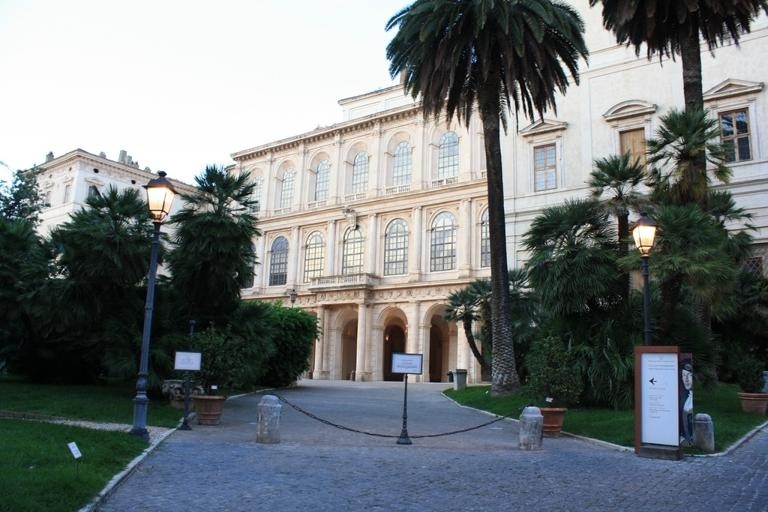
xmin=730 ymin=355 xmax=767 ymax=416
xmin=519 ymin=336 xmax=586 ymax=440
xmin=161 ymin=368 xmax=205 ymax=411
xmin=169 ymin=317 xmax=258 ymax=427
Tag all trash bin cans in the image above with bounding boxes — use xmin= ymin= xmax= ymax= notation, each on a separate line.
xmin=453 ymin=369 xmax=467 ymax=390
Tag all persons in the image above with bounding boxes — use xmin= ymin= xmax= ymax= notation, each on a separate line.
xmin=680 ymin=364 xmax=694 ymax=448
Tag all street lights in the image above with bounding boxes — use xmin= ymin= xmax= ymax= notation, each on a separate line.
xmin=627 ymin=207 xmax=659 ymax=344
xmin=123 ymin=168 xmax=178 ymax=438
xmin=288 ymin=288 xmax=297 ymax=309
